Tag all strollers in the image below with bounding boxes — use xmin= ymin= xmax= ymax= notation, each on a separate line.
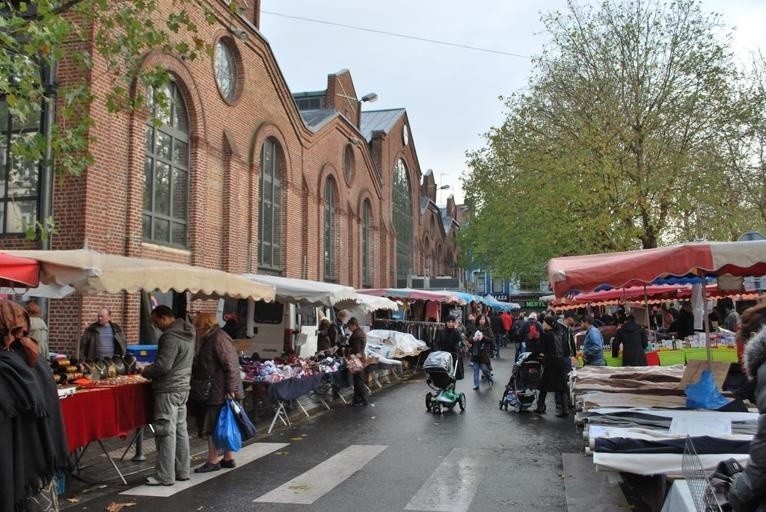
xmin=499 ymin=349 xmax=547 ymax=416
xmin=423 ymin=350 xmax=467 ymax=414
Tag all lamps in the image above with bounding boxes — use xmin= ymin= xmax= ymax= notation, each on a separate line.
xmin=432 ymin=183 xmax=452 ymax=195
xmin=226 ymin=0 xmax=250 ymax=39
xmin=344 ymin=91 xmax=379 ymax=112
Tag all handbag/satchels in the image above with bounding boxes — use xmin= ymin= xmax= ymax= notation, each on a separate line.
xmin=231 ymin=401 xmax=257 ymax=441
xmin=189 ymin=377 xmax=218 ymax=402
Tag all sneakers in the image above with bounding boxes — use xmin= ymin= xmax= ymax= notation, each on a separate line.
xmin=195 ymin=463 xmax=221 ymax=473
xmin=145 ymin=477 xmax=173 ymax=486
xmin=219 ymin=458 xmax=235 ymax=468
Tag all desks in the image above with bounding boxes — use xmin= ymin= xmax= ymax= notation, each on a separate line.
xmin=569 ymin=364 xmax=758 ymax=511
xmin=58 ymin=373 xmax=152 ymax=488
xmin=239 ymin=362 xmax=399 ymax=436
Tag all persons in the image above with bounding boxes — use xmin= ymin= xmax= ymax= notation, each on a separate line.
xmin=593 ymin=311 xmax=629 ymax=327
xmin=612 ymin=316 xmax=649 ymax=366
xmin=530 ymin=317 xmax=574 ymax=418
xmin=727 ymin=300 xmax=765 ymax=512
xmin=1 ymin=298 xmax=68 ymax=511
xmin=317 ymin=309 xmax=369 ymax=406
xmin=647 ymin=298 xmax=757 ymax=340
xmin=141 ymin=305 xmax=196 ymax=486
xmin=469 ymin=314 xmax=496 ymax=391
xmin=577 ymin=315 xmax=607 ymax=366
xmin=194 ymin=313 xmax=243 ymax=474
xmin=436 ymin=315 xmax=466 ymax=411
xmin=492 ymin=309 xmax=581 ymax=358
xmin=79 ymin=308 xmax=127 ymax=362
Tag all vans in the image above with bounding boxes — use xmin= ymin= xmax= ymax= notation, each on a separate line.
xmin=216 ymin=298 xmax=349 ymax=365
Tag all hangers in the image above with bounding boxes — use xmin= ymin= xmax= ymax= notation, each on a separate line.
xmin=405 ymin=320 xmax=445 ymax=330
